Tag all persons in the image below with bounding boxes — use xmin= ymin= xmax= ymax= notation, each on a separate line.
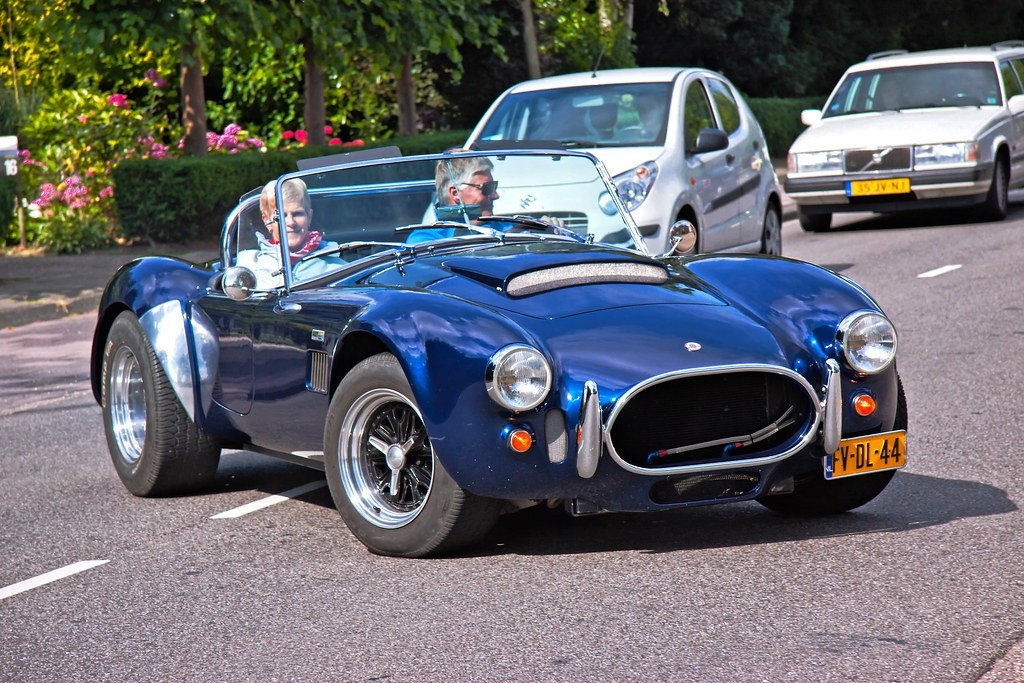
xmin=406 ymin=148 xmax=568 ymax=244
xmin=882 ymin=79 xmax=901 ymax=109
xmin=236 ymin=178 xmax=350 ymax=288
xmin=633 ymin=94 xmax=665 ymax=134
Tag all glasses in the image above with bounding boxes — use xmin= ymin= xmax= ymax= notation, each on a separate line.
xmin=459 ymin=182 xmax=498 ymax=196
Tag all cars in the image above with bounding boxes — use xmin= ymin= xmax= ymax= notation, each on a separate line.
xmin=422 ymin=67 xmax=783 ymax=264
xmin=786 ymin=39 xmax=1024 ymax=232
xmin=91 ymin=148 xmax=907 ymax=556
